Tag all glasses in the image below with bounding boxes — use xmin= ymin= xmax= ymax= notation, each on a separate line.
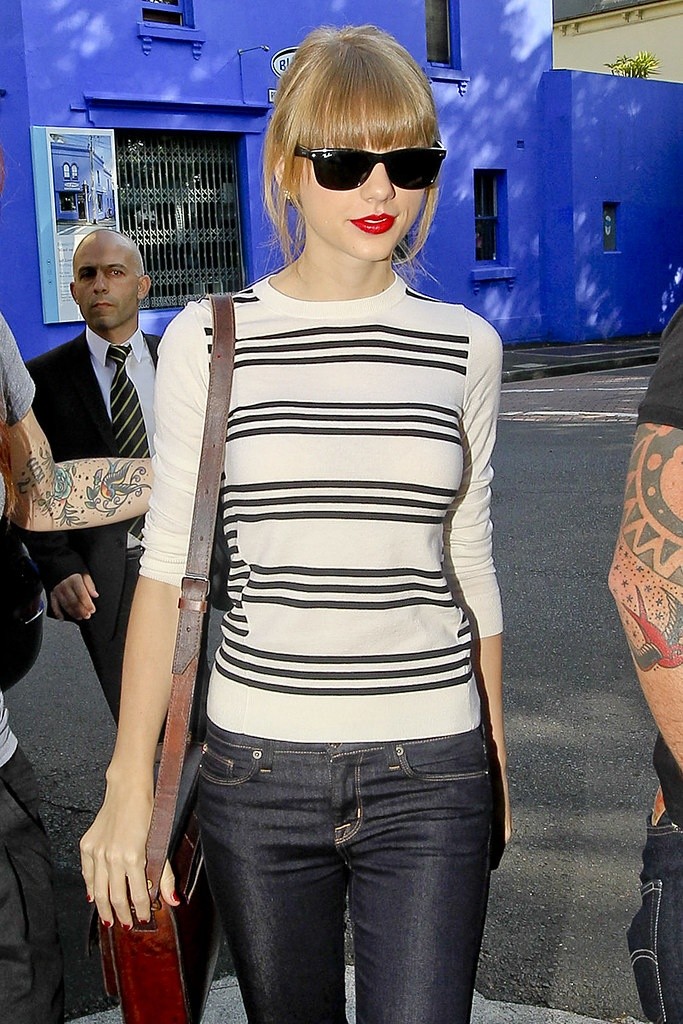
xmin=293 ymin=139 xmax=447 ymax=190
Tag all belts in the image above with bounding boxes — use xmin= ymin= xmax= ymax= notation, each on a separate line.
xmin=126 ymin=547 xmax=146 ymax=561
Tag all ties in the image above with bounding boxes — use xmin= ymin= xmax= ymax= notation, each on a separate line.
xmin=106 ymin=344 xmax=150 ymax=541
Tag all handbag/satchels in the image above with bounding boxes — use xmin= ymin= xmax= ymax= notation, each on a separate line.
xmin=0 ymin=520 xmax=44 ymax=693
xmin=86 ymin=740 xmax=223 ymax=1024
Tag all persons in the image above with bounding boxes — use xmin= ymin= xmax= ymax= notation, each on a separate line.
xmin=78 ymin=24 xmax=512 ymax=1024
xmin=0 ymin=317 xmax=158 ymax=1024
xmin=24 ymin=229 xmax=168 ymax=726
xmin=606 ymin=298 xmax=683 ymax=1024
